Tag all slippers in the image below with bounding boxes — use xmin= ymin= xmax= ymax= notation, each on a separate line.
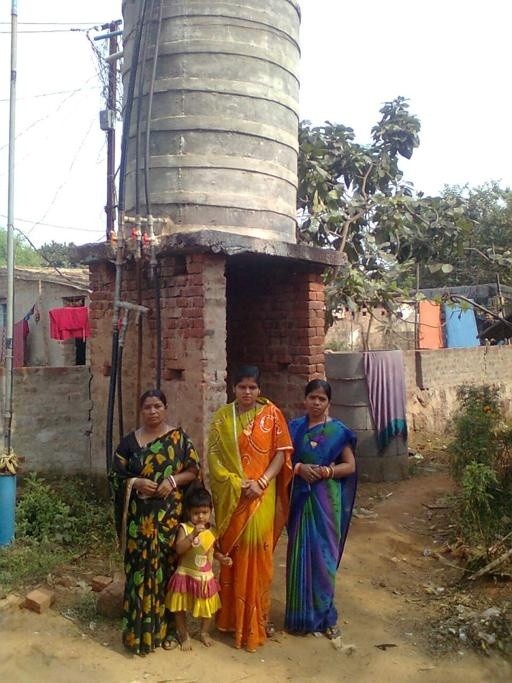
xmin=265 ymin=621 xmax=275 ymax=636
xmin=163 ymin=627 xmax=179 ymax=649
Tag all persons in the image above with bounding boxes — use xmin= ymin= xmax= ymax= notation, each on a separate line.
xmin=206 ymin=363 xmax=295 ymax=653
xmin=108 ymin=388 xmax=203 ymax=657
xmin=164 ymin=485 xmax=233 ymax=651
xmin=286 ymin=377 xmax=358 ymax=639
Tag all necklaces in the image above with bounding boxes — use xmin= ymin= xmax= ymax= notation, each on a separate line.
xmin=305 ymin=413 xmax=328 ymax=448
xmin=236 ymin=401 xmax=258 ymax=436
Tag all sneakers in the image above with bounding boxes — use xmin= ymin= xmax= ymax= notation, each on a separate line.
xmin=326 ymin=626 xmax=342 ymax=639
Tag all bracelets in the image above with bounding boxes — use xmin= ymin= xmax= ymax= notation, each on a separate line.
xmin=167 ymin=476 xmax=176 ymax=491
xmin=318 ymin=464 xmax=335 ymax=478
xmin=169 ymin=474 xmax=178 ymax=487
xmin=257 ymin=473 xmax=270 ymax=489
